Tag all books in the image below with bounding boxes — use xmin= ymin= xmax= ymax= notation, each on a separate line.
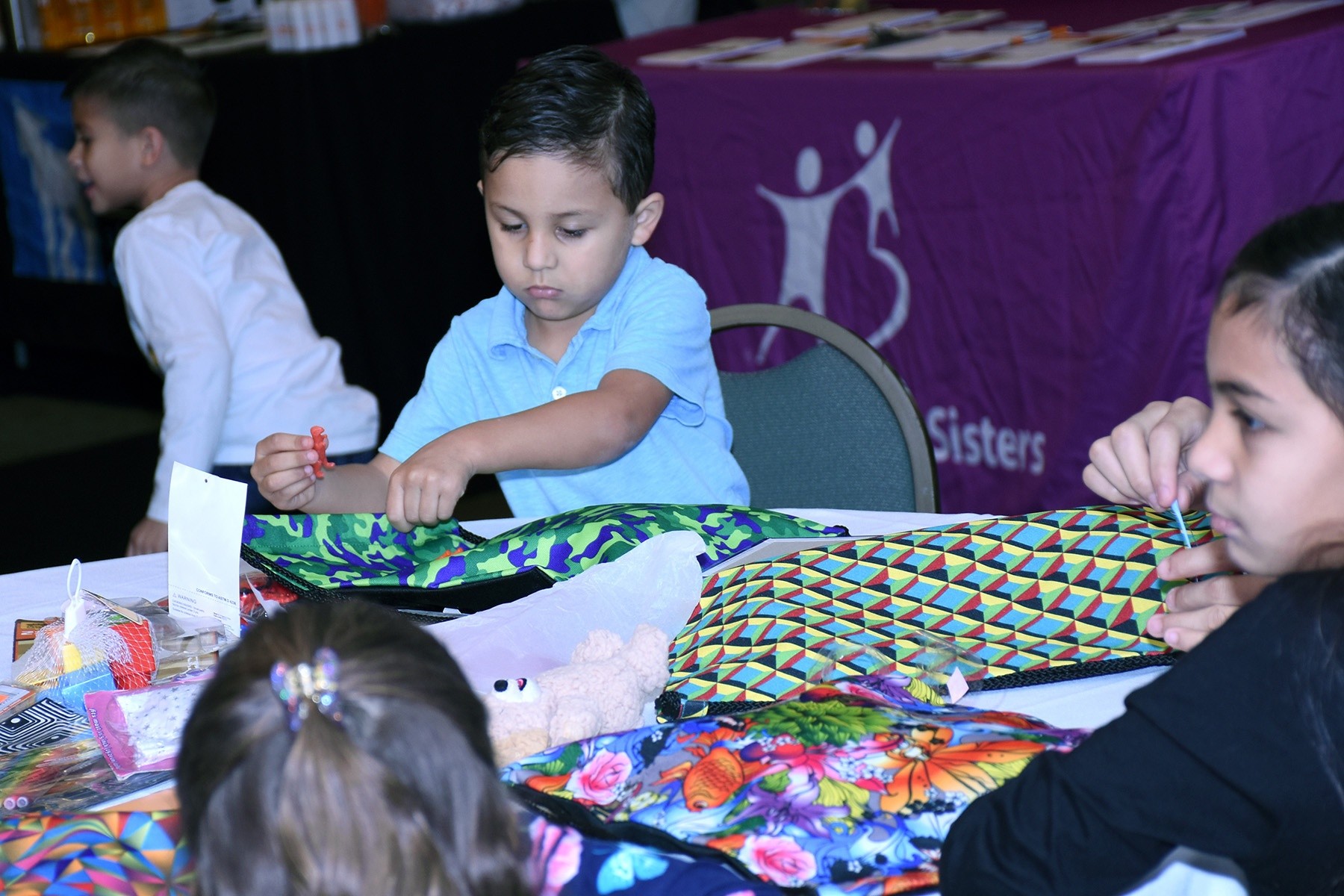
xmin=639 ymin=0 xmax=1344 ymax=68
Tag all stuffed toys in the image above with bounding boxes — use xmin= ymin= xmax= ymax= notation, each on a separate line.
xmin=479 ymin=627 xmax=670 ymax=770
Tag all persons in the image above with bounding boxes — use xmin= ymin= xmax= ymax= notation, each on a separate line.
xmin=59 ymin=38 xmax=379 ymax=558
xmin=938 ymin=200 xmax=1344 ymax=896
xmin=173 ymin=600 xmax=535 ymax=896
xmin=253 ymin=44 xmax=749 ymax=533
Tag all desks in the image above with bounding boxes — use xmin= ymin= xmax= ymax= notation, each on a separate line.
xmin=0 ymin=507 xmax=1262 ymax=896
xmin=614 ymin=0 xmax=1344 ymax=518
xmin=0 ymin=0 xmax=598 ymax=385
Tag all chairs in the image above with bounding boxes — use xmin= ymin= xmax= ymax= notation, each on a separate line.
xmin=708 ymin=303 xmax=935 ymax=515
